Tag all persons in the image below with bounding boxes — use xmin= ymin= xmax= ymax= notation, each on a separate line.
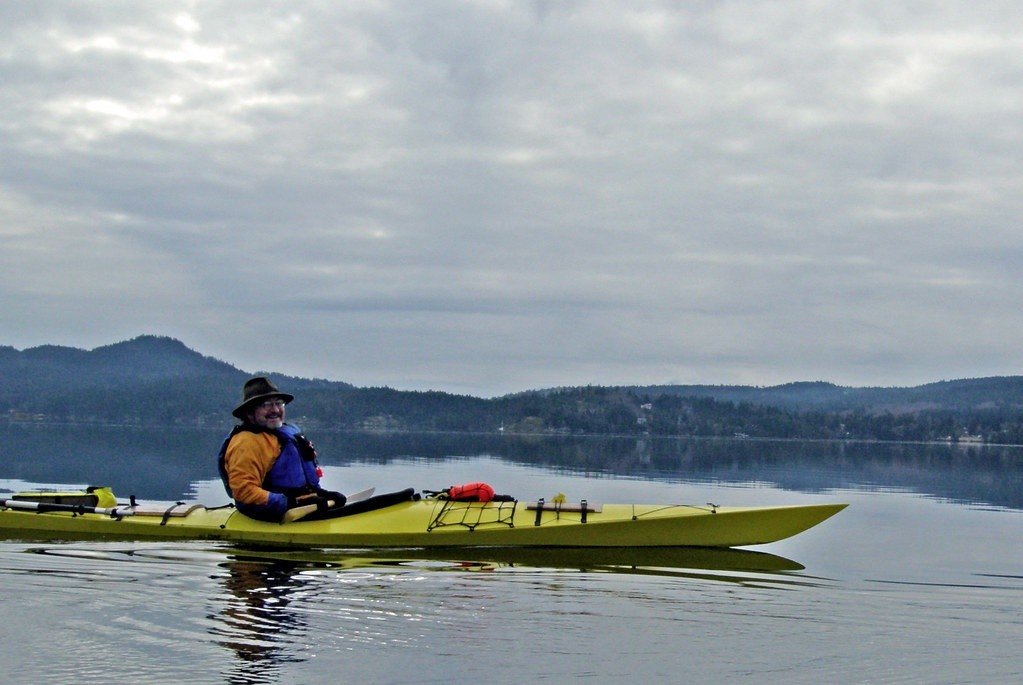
xmin=217 ymin=377 xmax=346 ymax=522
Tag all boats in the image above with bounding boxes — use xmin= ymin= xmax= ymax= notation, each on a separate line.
xmin=0 ymin=480 xmax=851 ymax=549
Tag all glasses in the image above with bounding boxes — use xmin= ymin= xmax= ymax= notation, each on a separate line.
xmin=256 ymin=399 xmax=286 ymax=409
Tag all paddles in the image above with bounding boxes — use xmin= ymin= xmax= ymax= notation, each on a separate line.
xmin=1 ymin=498 xmax=206 ymax=517
xmin=286 ymin=486 xmax=376 ymax=522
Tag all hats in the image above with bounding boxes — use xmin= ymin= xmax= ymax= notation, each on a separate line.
xmin=232 ymin=376 xmax=294 ymax=419
